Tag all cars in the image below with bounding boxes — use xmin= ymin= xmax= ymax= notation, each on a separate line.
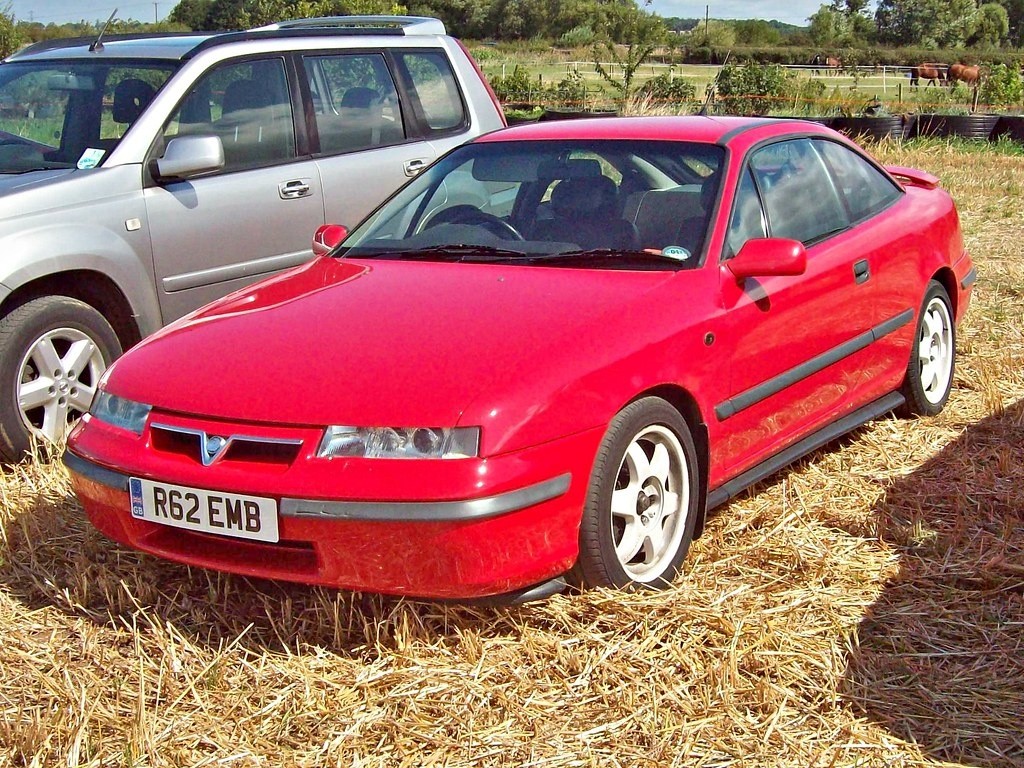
xmin=61 ymin=116 xmax=978 ymax=604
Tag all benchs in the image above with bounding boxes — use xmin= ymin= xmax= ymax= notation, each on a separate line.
xmin=265 ymin=86 xmax=405 ymax=152
xmin=620 ymin=188 xmax=836 ymax=249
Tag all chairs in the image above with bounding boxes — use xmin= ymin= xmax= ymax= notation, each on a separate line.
xmin=530 ymin=176 xmax=644 ymax=249
xmin=92 ymin=78 xmax=156 ymax=160
xmin=204 ymin=79 xmax=279 ymax=162
xmin=675 ymin=170 xmax=718 ymax=251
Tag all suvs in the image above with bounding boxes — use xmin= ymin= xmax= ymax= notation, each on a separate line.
xmin=0 ymin=17 xmax=517 ymax=475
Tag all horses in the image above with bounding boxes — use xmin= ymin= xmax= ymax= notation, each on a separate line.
xmin=909 ymin=63 xmax=981 ymax=93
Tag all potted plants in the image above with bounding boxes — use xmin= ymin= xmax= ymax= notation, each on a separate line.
xmin=742 ymin=62 xmax=1024 ymax=143
xmin=502 ymin=100 xmax=617 ymax=127
